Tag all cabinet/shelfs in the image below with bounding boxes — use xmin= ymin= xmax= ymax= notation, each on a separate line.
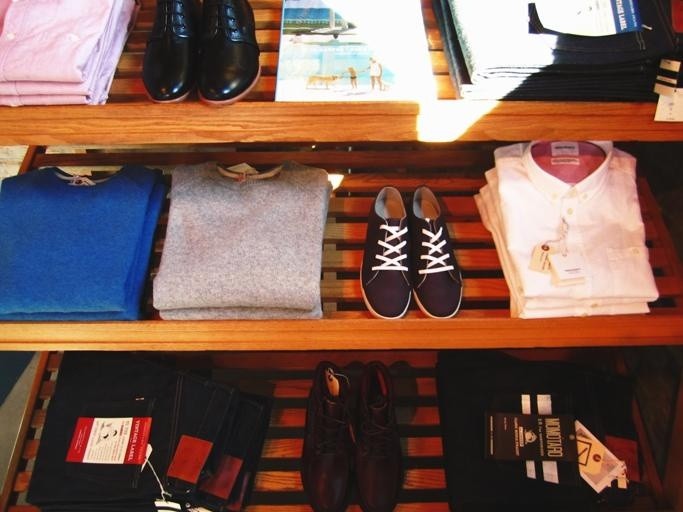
xmin=0 ymin=0 xmax=683 ymax=512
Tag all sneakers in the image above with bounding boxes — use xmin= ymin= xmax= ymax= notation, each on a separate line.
xmin=411 ymin=185 xmax=463 ymax=319
xmin=360 ymin=185 xmax=411 ymax=320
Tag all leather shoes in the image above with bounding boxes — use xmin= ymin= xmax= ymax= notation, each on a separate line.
xmin=197 ymin=0 xmax=260 ymax=105
xmin=301 ymin=360 xmax=354 ymax=512
xmin=354 ymin=361 xmax=404 ymax=512
xmin=141 ymin=1 xmax=197 ymax=104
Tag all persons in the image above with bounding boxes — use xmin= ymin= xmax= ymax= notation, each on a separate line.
xmin=364 ymin=56 xmax=383 ymax=91
xmin=345 ymin=67 xmax=365 ymax=89
